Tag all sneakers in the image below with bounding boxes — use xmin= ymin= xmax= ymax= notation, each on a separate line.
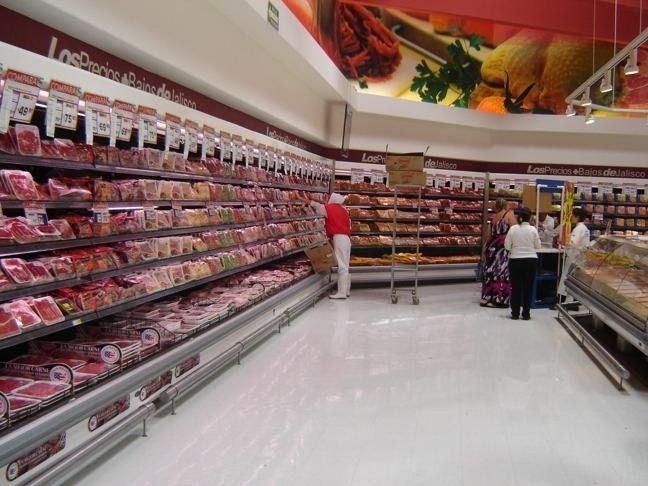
xmin=329 ymin=293 xmax=350 ymax=299
xmin=512 ymin=314 xmax=531 ymax=320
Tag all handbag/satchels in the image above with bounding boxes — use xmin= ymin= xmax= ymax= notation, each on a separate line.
xmin=474 ymin=261 xmax=483 ymax=281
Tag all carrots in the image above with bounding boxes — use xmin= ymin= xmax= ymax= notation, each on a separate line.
xmin=475 ymin=97 xmax=532 ymax=114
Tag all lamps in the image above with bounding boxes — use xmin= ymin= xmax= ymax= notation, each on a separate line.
xmin=564 ymin=0 xmax=648 ymax=124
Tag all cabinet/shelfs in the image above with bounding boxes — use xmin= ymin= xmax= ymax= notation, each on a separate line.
xmin=0 ymin=154 xmax=648 ymax=486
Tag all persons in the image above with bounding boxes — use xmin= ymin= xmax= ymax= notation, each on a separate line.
xmin=479 ymin=199 xmax=516 ymax=308
xmin=504 ymin=207 xmax=541 ymax=320
xmin=295 ymin=190 xmax=352 ymax=299
xmin=557 ymin=208 xmax=590 ymax=310
xmin=538 ymin=209 xmax=560 ymax=249
